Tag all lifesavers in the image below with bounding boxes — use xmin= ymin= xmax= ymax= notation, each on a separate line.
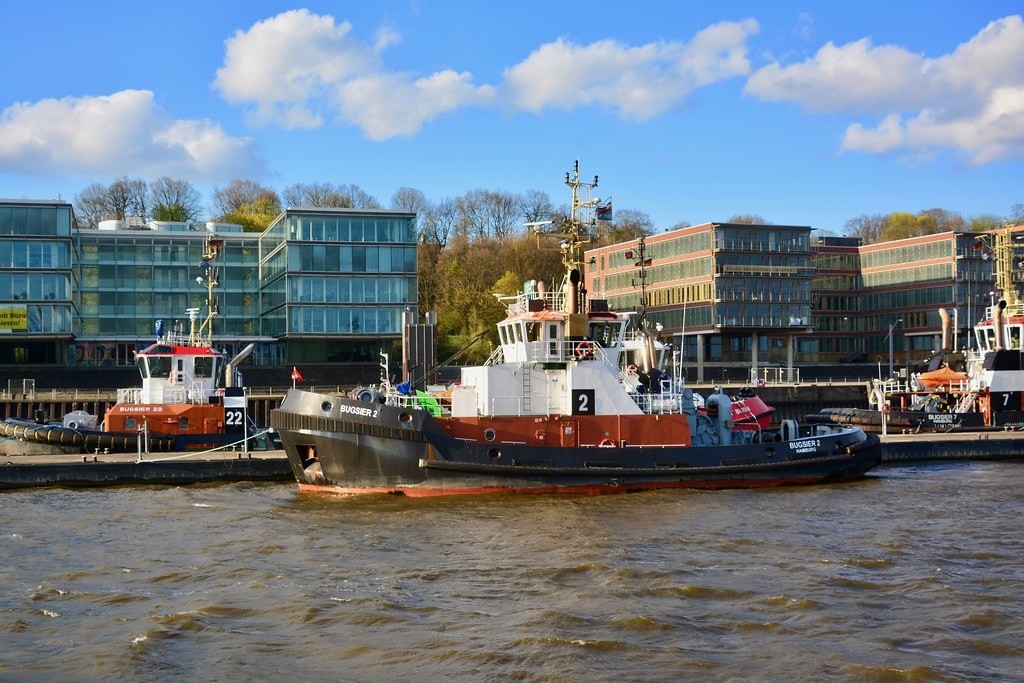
xmin=627 ymin=363 xmax=638 ymax=375
xmin=882 ymin=403 xmax=892 ymax=414
xmin=936 ymin=401 xmax=950 ymax=413
xmin=576 ymin=342 xmax=593 ymax=356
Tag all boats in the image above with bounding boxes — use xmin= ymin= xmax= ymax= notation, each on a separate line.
xmin=805 ymin=219 xmax=1024 ymax=431
xmin=1 ymin=232 xmax=303 ymax=486
xmin=270 ymin=153 xmax=882 ymax=499
